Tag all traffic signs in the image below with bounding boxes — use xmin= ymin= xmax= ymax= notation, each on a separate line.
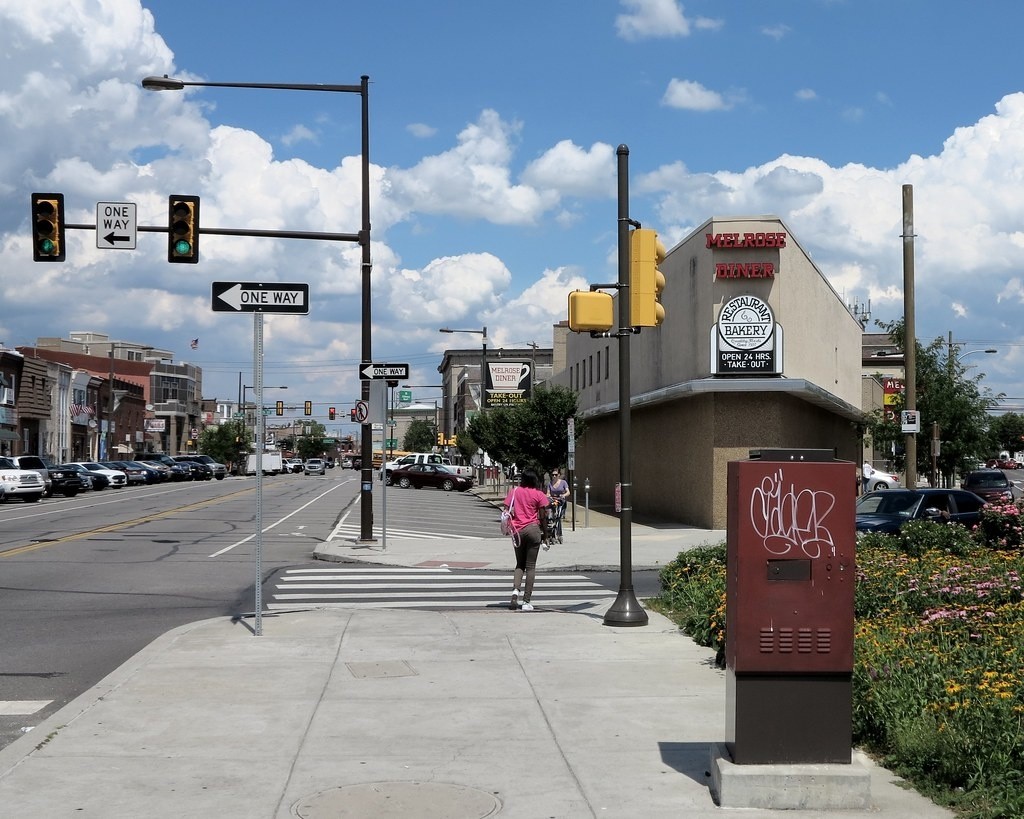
xmin=96 ymin=200 xmax=137 ymax=251
xmin=358 ymin=363 xmax=410 ymax=380
xmin=210 ymin=280 xmax=311 ymax=316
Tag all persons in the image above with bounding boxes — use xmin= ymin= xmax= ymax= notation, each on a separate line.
xmin=504 ymin=471 xmax=550 ymax=611
xmin=546 ymin=469 xmax=570 ymax=537
xmin=863 ymin=460 xmax=872 ymax=493
xmin=925 ymin=507 xmax=951 ymax=519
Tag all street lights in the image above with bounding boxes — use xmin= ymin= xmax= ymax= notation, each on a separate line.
xmin=106 ymin=341 xmax=155 ymax=461
xmin=402 ymin=385 xmax=449 ymax=444
xmin=438 ymin=327 xmax=486 ymax=487
xmin=140 ymin=73 xmax=374 ymax=540
xmin=415 ymin=401 xmax=437 ymax=445
xmin=242 ymin=384 xmax=288 ymax=444
xmin=948 ymin=330 xmax=997 ymax=390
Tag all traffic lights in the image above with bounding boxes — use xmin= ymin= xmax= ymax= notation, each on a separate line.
xmin=628 ymin=228 xmax=666 ymax=328
xmin=351 ymin=409 xmax=356 ymax=422
xmin=329 ymin=407 xmax=335 ymax=420
xmin=167 ymin=194 xmax=199 ymax=265
xmin=31 ymin=193 xmax=66 ymax=262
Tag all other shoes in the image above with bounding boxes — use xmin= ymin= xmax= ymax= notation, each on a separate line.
xmin=522 ymin=604 xmax=533 ymax=611
xmin=511 ymin=589 xmax=519 ymax=609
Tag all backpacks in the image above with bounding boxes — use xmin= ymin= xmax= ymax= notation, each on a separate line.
xmin=500 ymin=487 xmax=521 ymax=547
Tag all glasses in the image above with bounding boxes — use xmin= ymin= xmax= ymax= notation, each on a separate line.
xmin=550 ymin=474 xmax=558 ymax=476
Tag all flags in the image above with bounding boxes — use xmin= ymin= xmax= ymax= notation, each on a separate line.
xmin=80 ymin=403 xmax=96 ymax=414
xmin=69 ymin=403 xmax=82 ymax=417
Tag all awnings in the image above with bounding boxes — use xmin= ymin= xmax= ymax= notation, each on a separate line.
xmin=0 ymin=429 xmax=21 ymax=441
xmin=118 ymin=444 xmax=133 ymax=454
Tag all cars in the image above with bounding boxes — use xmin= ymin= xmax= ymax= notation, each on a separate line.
xmin=61 ymin=452 xmax=213 ymax=492
xmin=331 ymin=452 xmax=474 ymax=494
xmin=855 ymin=459 xmax=1018 ymax=540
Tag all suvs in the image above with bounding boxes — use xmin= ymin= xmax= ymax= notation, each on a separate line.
xmin=282 ymin=458 xmax=302 ymax=474
xmin=5 ymin=456 xmax=52 ymax=497
xmin=305 ymin=458 xmax=325 ymax=475
xmin=171 ymin=454 xmax=226 ymax=481
xmin=0 ymin=456 xmax=46 ymax=502
xmin=40 ymin=458 xmax=81 ymax=498
